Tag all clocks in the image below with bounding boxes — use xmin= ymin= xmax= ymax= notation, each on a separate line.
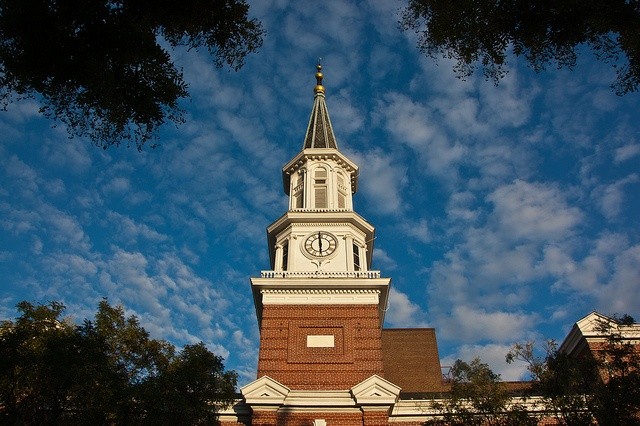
xmin=300 ymin=230 xmax=341 ymax=260
